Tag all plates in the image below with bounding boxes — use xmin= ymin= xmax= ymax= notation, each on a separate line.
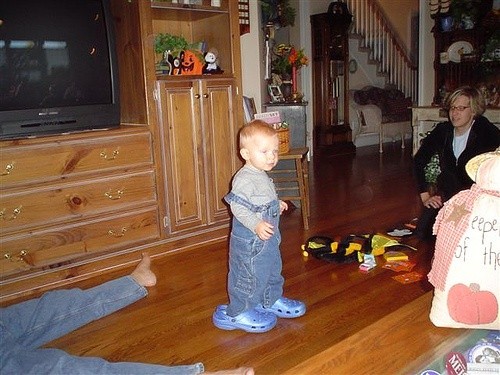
xmin=448 ymin=41 xmax=473 ymax=62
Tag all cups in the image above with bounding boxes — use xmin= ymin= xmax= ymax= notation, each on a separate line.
xmin=440 ymin=16 xmax=455 ymax=31
xmin=440 ymin=53 xmax=449 ymax=64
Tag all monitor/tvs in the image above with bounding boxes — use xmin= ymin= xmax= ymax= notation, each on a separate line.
xmin=0 ymin=0 xmax=120 ymax=139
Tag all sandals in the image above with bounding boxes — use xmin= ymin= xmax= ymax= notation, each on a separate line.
xmin=213 ymin=304 xmax=278 ymax=333
xmin=256 ymin=296 xmax=306 ymax=319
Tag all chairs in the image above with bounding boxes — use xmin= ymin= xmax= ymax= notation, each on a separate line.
xmin=348 ymin=85 xmax=413 ymax=153
xmin=242 ymin=95 xmax=311 ymax=230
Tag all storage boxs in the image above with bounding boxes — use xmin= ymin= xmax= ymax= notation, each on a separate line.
xmin=383 ymin=251 xmax=408 ymax=263
xmin=253 ymin=111 xmax=282 ymax=125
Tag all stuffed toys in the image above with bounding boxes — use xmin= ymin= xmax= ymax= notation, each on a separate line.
xmin=201 ymin=51 xmax=224 ymax=75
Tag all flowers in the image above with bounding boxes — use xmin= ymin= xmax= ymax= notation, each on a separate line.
xmin=271 ymin=44 xmax=309 ymax=77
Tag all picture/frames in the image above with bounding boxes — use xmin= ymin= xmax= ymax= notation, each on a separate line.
xmin=269 ymin=85 xmax=285 ymax=102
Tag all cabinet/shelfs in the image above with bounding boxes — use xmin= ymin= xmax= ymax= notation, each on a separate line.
xmin=412 ymin=107 xmax=500 ymax=157
xmin=430 ymin=0 xmax=500 ymax=109
xmin=0 ymin=122 xmax=169 ymax=305
xmin=309 ymin=1 xmax=353 ymax=149
xmin=114 ymin=0 xmax=246 ymax=256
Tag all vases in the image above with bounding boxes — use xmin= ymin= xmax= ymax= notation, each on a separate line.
xmin=280 ymin=80 xmax=292 ymax=99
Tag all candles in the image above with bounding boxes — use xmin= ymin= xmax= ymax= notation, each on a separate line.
xmin=292 ymin=65 xmax=297 ymax=94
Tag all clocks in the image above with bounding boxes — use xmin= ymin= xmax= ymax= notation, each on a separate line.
xmin=349 ymin=58 xmax=358 ymax=74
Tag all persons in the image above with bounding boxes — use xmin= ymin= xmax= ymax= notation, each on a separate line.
xmin=475 ymin=348 xmax=500 ymax=363
xmin=0 ymin=252 xmax=256 ymax=374
xmin=213 ymin=118 xmax=306 ymax=333
xmin=410 ymin=84 xmax=500 ymax=241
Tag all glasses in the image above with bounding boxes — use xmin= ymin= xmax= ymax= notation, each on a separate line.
xmin=447 ymin=105 xmax=471 ymax=111
